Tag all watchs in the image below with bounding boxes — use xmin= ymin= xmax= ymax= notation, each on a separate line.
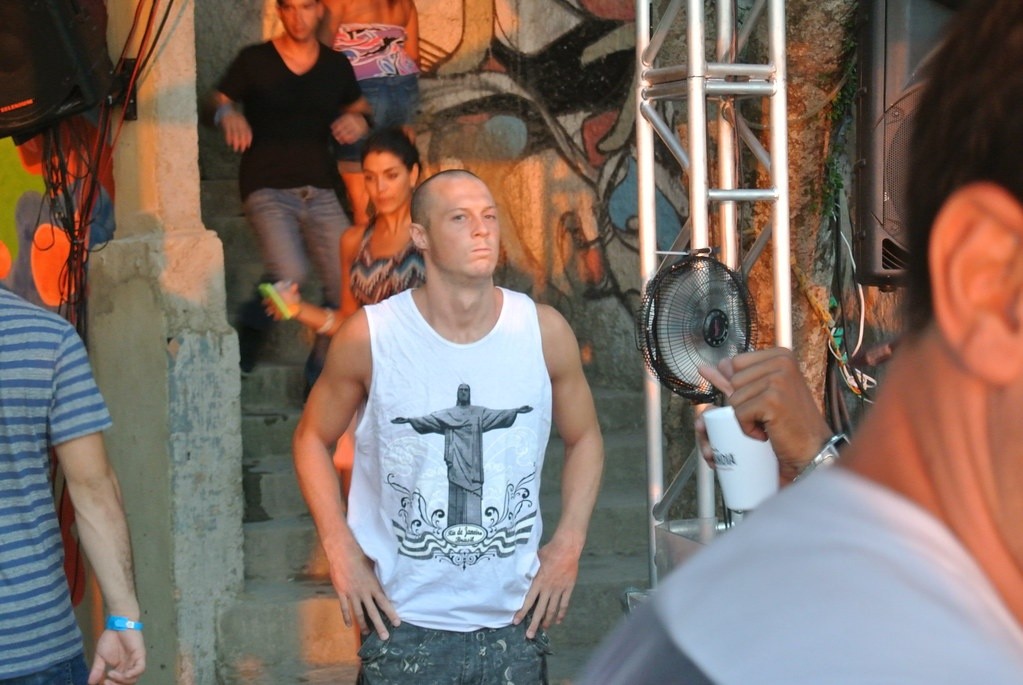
xmin=794 ymin=433 xmax=852 ymax=489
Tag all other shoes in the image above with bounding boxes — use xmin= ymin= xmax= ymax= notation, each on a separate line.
xmin=235 ymin=311 xmax=268 ymax=376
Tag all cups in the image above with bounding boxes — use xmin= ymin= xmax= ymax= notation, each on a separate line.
xmin=704 ymin=404 xmax=780 ymax=511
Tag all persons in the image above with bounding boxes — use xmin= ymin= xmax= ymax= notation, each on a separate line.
xmin=0 ymin=280 xmax=146 ymax=684
xmin=577 ymin=1 xmax=1023 ymax=685
xmin=214 ymin=3 xmax=424 ymax=582
xmin=294 ymin=169 xmax=604 ymax=685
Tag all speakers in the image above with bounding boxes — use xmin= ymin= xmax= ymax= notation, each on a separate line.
xmin=850 ymin=0 xmax=965 ymax=293
xmin=0 ymin=0 xmax=103 ymax=139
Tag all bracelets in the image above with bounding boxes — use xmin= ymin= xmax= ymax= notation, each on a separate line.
xmin=317 ymin=306 xmax=335 ymax=336
xmin=217 ymin=104 xmax=244 ymax=118
xmin=101 ymin=613 xmax=143 ymax=634
